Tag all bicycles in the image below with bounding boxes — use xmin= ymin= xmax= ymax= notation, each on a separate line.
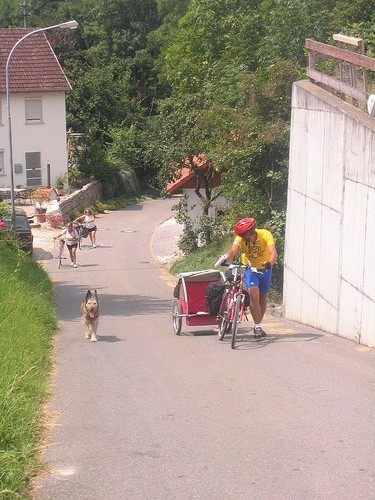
xmin=72 ymin=221 xmax=87 ymax=249
xmin=215 ymin=260 xmax=266 ymax=349
xmin=53 ymin=237 xmax=68 ymax=269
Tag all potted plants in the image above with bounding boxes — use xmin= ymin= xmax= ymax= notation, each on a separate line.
xmin=30 ymin=190 xmax=50 ymax=222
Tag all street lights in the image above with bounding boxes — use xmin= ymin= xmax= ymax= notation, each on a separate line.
xmin=5 ymin=18 xmax=80 ymax=239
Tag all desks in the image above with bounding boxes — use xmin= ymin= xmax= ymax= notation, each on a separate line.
xmin=0 ymin=188 xmax=28 ymax=206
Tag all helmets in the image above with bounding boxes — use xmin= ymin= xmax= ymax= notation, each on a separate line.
xmin=235 ymin=217 xmax=257 ymax=238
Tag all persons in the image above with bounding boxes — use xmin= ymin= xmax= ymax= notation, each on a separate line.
xmin=223 ymin=217 xmax=277 ymax=339
xmin=73 ymin=209 xmax=97 ymax=248
xmin=54 ymin=222 xmax=79 ymax=268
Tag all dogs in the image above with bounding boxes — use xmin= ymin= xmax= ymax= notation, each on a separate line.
xmin=80 ymin=290 xmax=102 ymax=342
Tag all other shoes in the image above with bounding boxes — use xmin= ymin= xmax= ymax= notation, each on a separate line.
xmin=261 ymin=329 xmax=267 ymax=337
xmin=73 ymin=264 xmax=77 ymax=268
xmin=70 ymin=262 xmax=73 ymax=265
xmin=254 ymin=327 xmax=262 ymax=338
xmin=93 ymin=244 xmax=96 ymax=248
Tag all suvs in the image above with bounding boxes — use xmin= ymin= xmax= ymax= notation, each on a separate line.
xmin=1 ymin=207 xmax=34 ymax=255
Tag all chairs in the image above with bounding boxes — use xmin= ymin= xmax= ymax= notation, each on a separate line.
xmin=180 ymin=281 xmax=222 ymax=317
xmin=0 ymin=185 xmax=29 ymax=205
